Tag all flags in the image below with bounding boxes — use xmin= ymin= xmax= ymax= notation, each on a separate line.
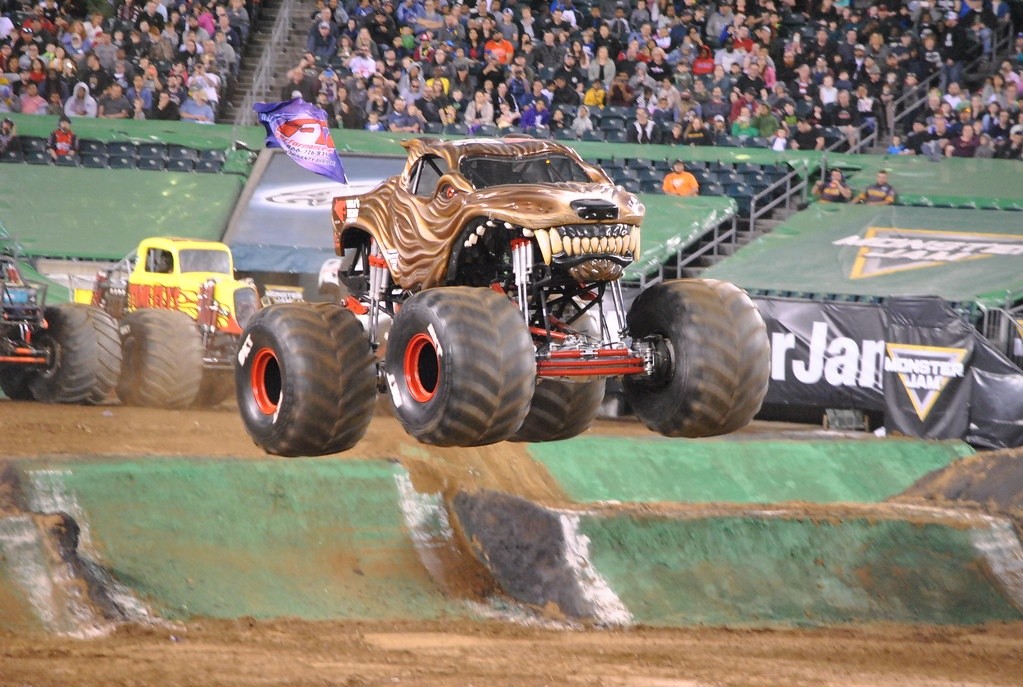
xmin=252 ymin=97 xmax=347 ymax=185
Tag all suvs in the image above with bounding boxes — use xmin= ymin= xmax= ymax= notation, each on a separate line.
xmin=80 ymin=233 xmax=288 ymax=409
xmin=0 ymin=225 xmax=125 ymax=409
xmin=229 ymin=136 xmax=775 ymax=455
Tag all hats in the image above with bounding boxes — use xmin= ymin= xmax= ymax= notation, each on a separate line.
xmin=0 ymin=0 xmax=1023 ymax=204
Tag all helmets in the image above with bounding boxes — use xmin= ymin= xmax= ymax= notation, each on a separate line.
xmin=467 ymin=159 xmax=499 ymax=186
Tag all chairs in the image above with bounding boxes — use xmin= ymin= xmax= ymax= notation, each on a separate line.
xmin=579 ymin=156 xmax=803 ymax=219
xmin=0 ymin=135 xmax=226 ymax=174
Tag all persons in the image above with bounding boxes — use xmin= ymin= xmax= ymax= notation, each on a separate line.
xmin=662 ymin=159 xmax=699 ymax=196
xmin=850 ymin=169 xmax=896 ymax=205
xmin=0 ymin=0 xmax=263 ymax=121
xmin=0 ymin=117 xmax=25 ymax=162
xmin=811 ymin=168 xmax=851 ymax=203
xmin=280 ymin=0 xmax=1023 ymax=163
xmin=45 ymin=114 xmax=81 ymax=167
